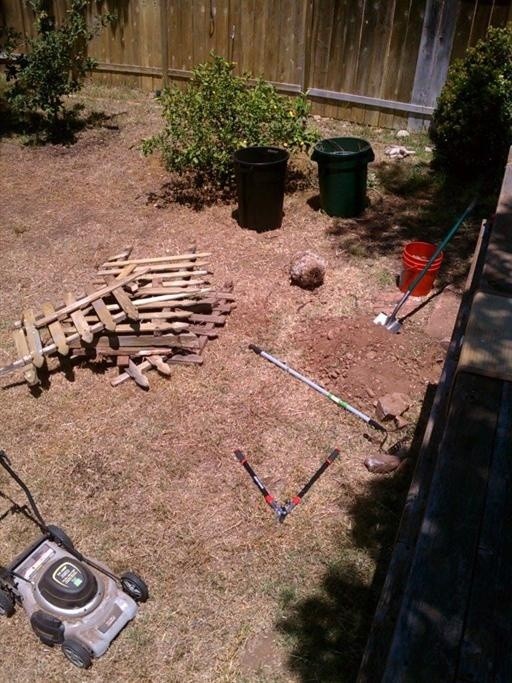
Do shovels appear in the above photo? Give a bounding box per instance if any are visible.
[372,200,478,333]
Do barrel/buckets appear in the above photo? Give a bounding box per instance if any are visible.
[399,240,444,297]
